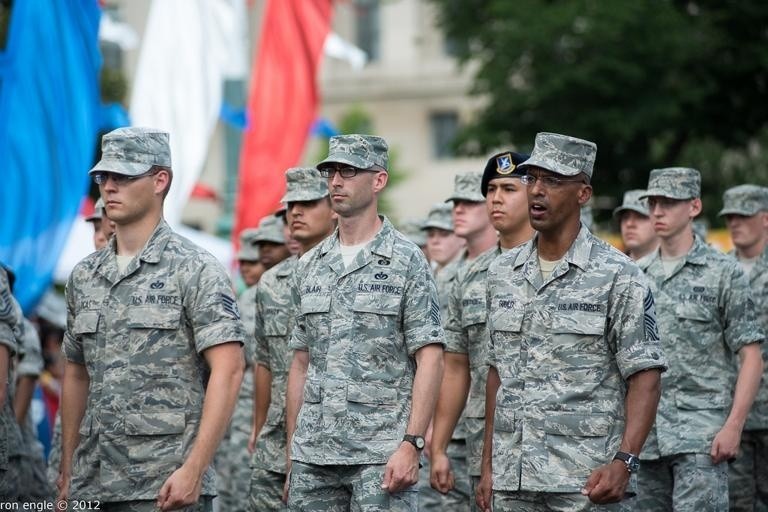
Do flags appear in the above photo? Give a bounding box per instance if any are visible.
[128,0,246,230]
[2,0,104,317]
[234,1,338,266]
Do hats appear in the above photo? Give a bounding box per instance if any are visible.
[279,166,330,203]
[315,134,390,171]
[88,126,172,177]
[85,197,104,221]
[420,204,454,232]
[638,167,702,199]
[444,170,486,203]
[250,214,284,244]
[236,228,260,262]
[398,218,427,247]
[516,133,597,179]
[613,189,650,217]
[274,205,288,216]
[481,152,529,197]
[716,181,767,217]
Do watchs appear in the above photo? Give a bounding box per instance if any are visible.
[402,434,426,451]
[612,451,641,473]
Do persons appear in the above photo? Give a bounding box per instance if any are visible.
[84,205,105,253]
[99,202,116,243]
[609,190,659,271]
[235,230,264,286]
[436,171,498,512]
[246,166,350,511]
[217,215,294,512]
[635,167,764,511]
[475,133,668,511]
[431,150,531,512]
[719,183,768,512]
[421,207,463,273]
[275,204,301,254]
[0,264,44,511]
[57,125,245,511]
[283,133,445,512]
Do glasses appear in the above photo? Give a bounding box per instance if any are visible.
[642,198,675,209]
[520,175,583,187]
[93,170,153,184]
[321,167,358,178]
